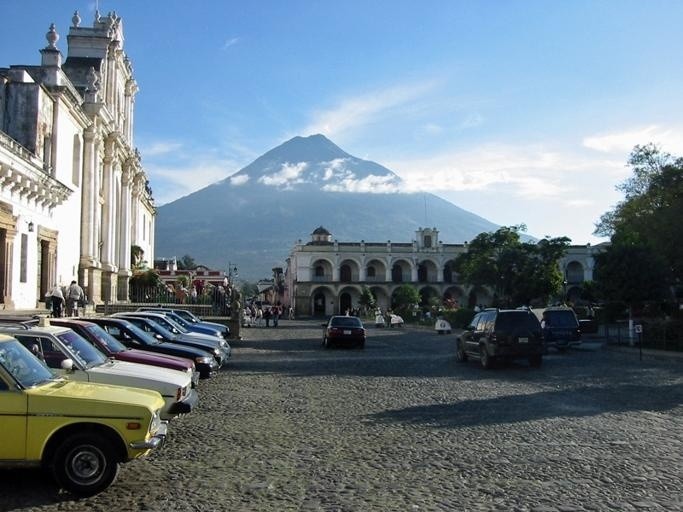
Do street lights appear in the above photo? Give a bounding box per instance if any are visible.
[562,280,568,305]
[499,272,505,302]
[227,263,238,287]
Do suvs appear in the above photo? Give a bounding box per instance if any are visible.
[455,306,542,368]
[253,299,281,316]
[526,303,583,353]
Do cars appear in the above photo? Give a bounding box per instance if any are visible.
[320,316,365,349]
[590,299,682,311]
[577,315,599,334]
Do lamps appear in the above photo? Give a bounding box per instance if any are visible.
[22,220,34,233]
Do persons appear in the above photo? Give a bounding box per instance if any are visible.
[45,286,65,318]
[66,281,83,317]
[346,306,393,327]
[246,303,294,327]
